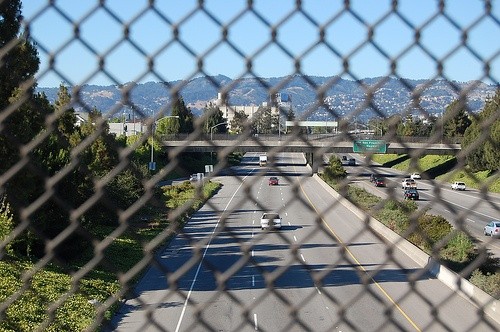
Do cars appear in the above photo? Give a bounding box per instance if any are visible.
[411,172,421,179]
[260,213,283,230]
[451,182,466,191]
[268,177,279,185]
[370,173,386,187]
[349,159,355,165]
[483,221,500,238]
[404,189,419,200]
[341,156,347,161]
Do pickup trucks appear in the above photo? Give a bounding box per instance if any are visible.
[401,178,417,189]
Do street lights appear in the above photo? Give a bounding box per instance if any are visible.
[210,121,228,164]
[150,115,182,170]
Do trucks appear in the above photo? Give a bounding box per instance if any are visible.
[259,154,267,167]
